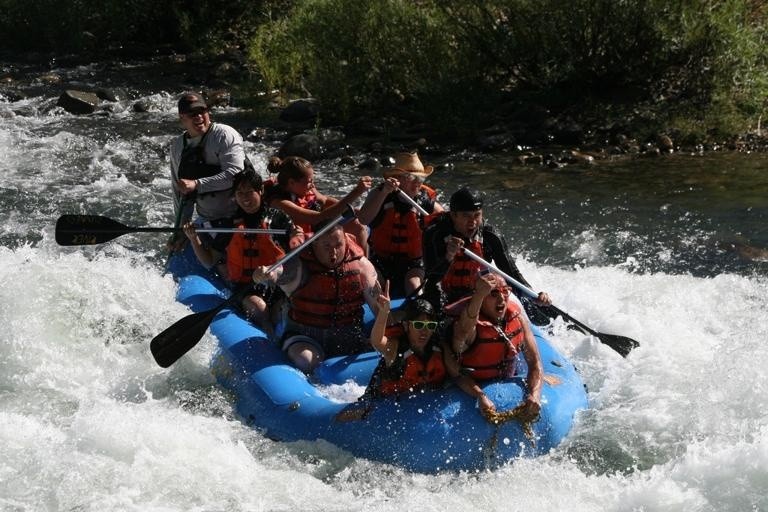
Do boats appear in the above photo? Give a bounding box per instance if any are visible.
[164,216,587,479]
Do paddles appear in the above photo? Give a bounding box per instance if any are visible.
[56,215,293,246]
[150,202,358,370]
[462,246,639,357]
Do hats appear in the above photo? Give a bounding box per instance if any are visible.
[449,185,483,212]
[383,153,433,178]
[178,93,209,113]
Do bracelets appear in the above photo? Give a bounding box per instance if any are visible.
[478,393,486,401]
[466,302,479,319]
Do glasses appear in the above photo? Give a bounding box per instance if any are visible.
[409,318,438,331]
[490,285,512,298]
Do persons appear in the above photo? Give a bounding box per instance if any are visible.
[165,92,255,255]
[251,218,392,372]
[264,157,372,233]
[421,186,553,309]
[362,279,447,401]
[450,268,543,419]
[359,152,443,278]
[182,170,307,332]
